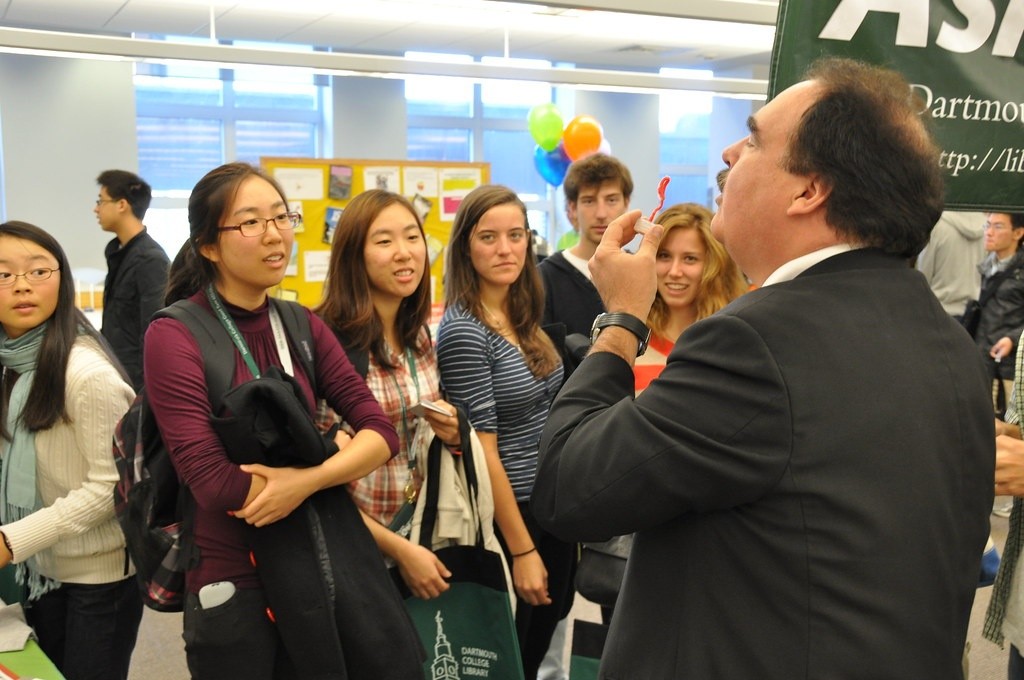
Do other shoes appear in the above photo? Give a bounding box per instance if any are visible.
[995,502,1016,518]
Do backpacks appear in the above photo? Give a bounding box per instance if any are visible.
[113,284,316,613]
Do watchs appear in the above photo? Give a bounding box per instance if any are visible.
[589,311,652,357]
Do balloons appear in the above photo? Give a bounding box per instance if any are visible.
[531,103,613,190]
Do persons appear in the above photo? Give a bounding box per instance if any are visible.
[915,211,987,323]
[981,328,1024,680]
[436,185,575,680]
[308,190,516,680]
[531,62,997,680]
[95,170,170,585]
[0,219,144,680]
[601,203,750,398]
[975,213,1024,421]
[535,153,634,376]
[145,162,427,680]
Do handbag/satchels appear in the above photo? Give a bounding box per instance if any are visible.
[960,299,983,335]
[414,408,525,680]
[575,524,634,605]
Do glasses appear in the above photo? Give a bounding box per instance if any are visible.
[98,196,123,206]
[0,266,61,289]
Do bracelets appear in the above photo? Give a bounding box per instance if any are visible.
[1,531,13,556]
[509,546,538,558]
